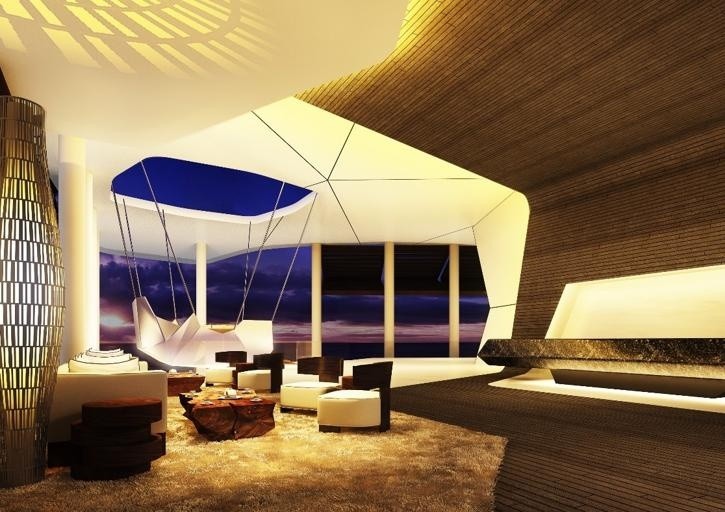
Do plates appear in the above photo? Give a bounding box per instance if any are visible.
[201,402,214,405]
[250,397,263,402]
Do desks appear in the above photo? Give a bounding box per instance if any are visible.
[178,392,273,439]
[167,374,205,396]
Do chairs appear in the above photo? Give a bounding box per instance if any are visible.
[317,361,392,432]
[236,352,283,390]
[205,351,247,387]
[279,356,344,415]
[46,348,167,467]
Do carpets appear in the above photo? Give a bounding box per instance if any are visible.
[0,390,510,512]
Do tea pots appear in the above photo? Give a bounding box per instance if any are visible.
[224,384,236,398]
